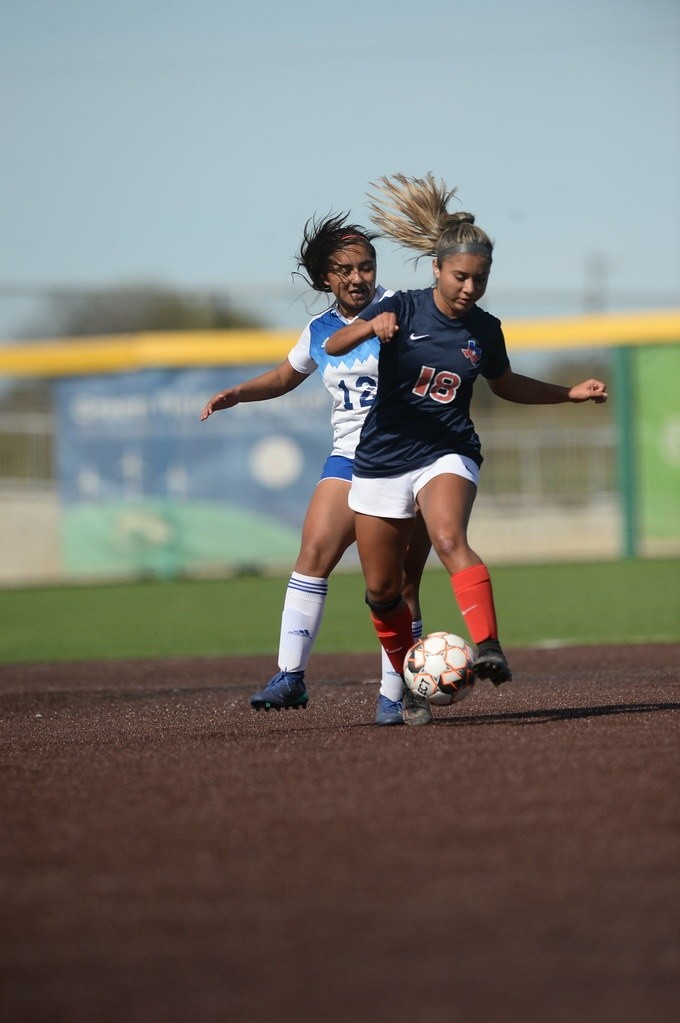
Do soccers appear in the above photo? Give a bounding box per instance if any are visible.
[404,630,479,708]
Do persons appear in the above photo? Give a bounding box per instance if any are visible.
[199,207,433,727]
[324,170,608,726]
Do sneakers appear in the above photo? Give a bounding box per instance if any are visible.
[375,694,403,727]
[471,647,514,687]
[401,685,432,726]
[249,670,308,712]
